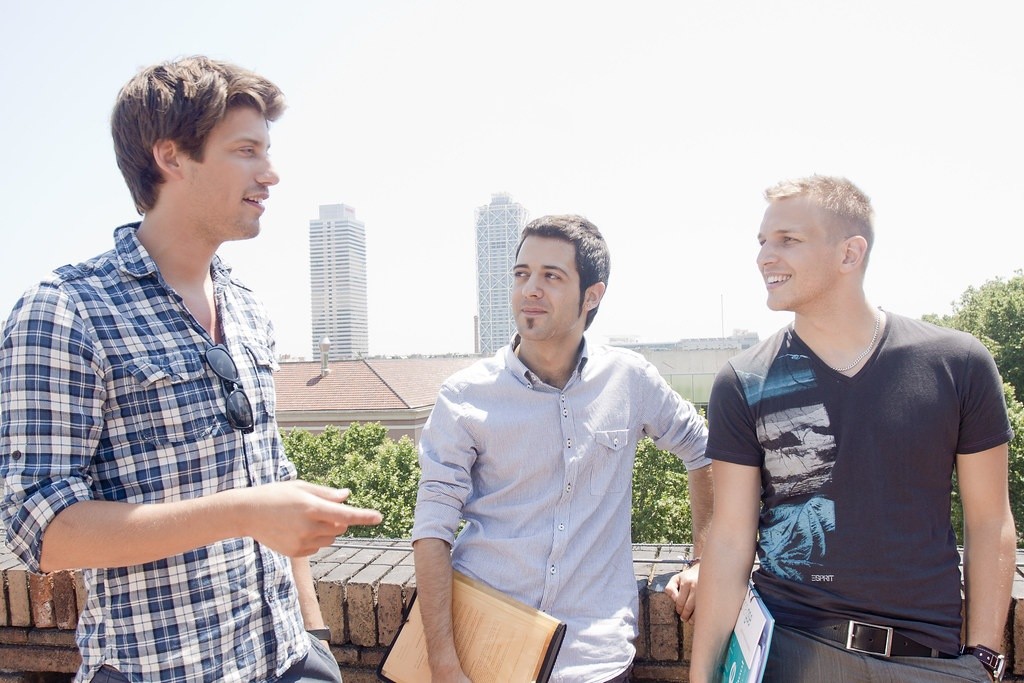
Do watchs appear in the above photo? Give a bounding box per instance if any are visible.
[960,644,1007,683]
[306,625,332,644]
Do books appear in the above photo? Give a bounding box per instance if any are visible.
[378,568,566,683]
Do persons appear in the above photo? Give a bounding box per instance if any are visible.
[412,211,719,683]
[0,54,382,683]
[689,174,1016,683]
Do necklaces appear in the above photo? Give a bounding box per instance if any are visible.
[832,304,881,371]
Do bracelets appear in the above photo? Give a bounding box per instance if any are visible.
[688,557,701,566]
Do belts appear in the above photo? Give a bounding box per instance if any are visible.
[764,603,960,660]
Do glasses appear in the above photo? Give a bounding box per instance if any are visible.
[204,344,255,433]
